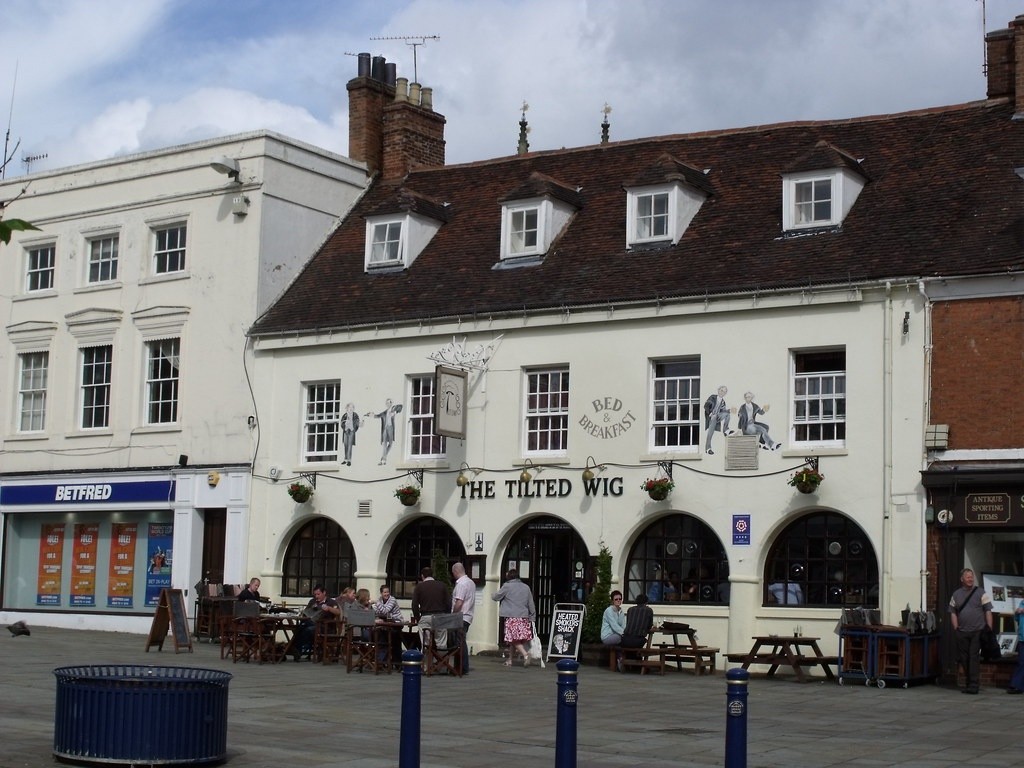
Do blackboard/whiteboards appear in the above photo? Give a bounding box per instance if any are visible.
[146,588,192,647]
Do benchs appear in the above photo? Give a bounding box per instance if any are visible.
[723,653,844,666]
[592,643,719,673]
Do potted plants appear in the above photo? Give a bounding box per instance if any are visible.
[579,539,617,666]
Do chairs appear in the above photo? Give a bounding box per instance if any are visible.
[216,599,465,678]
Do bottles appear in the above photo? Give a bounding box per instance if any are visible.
[266,601,271,612]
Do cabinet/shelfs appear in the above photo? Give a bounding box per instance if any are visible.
[200,596,232,636]
[844,625,936,677]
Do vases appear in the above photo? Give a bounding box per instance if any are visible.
[292,493,309,503]
[400,494,418,506]
[797,479,817,494]
[648,487,669,501]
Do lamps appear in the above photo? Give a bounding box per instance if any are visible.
[458,461,480,486]
[582,456,607,481]
[521,458,541,482]
[212,155,244,183]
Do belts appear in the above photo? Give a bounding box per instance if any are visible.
[421,611,446,616]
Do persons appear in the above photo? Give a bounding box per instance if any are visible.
[294,584,340,659]
[768,568,804,605]
[600,590,653,671]
[690,564,711,601]
[338,587,383,642]
[948,568,993,693]
[492,569,536,666]
[411,567,451,676]
[238,578,261,603]
[649,567,674,604]
[374,585,404,630]
[1007,599,1024,694]
[451,562,476,673]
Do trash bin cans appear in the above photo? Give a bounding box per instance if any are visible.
[51,664,234,768]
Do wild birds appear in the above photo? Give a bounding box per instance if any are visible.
[6,620,32,637]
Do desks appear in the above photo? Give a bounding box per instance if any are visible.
[741,637,834,682]
[358,622,419,675]
[258,614,311,663]
[640,627,702,674]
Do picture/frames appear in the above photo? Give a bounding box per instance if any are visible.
[998,632,1019,655]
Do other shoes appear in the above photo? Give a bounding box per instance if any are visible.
[502,658,512,666]
[450,666,469,675]
[523,654,530,667]
[617,656,626,671]
[961,688,978,694]
[1007,688,1023,694]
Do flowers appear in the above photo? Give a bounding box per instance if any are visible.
[287,483,313,496]
[392,485,421,498]
[788,468,824,487]
[640,477,675,492]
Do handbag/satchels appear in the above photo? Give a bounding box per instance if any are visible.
[522,621,542,660]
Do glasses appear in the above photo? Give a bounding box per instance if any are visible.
[615,599,622,601]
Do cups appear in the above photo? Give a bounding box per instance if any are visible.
[387,613,393,620]
[299,606,304,613]
[411,617,415,623]
